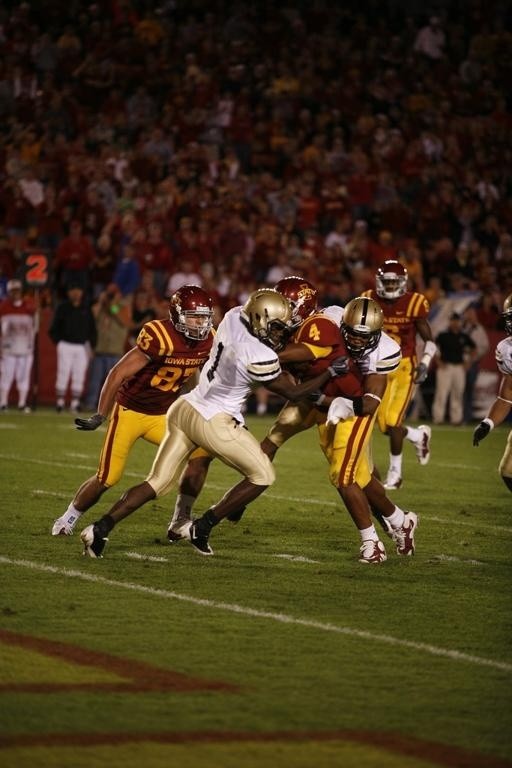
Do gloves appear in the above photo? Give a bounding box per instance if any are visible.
[329,355,354,378]
[413,362,428,384]
[472,422,490,446]
[75,414,107,431]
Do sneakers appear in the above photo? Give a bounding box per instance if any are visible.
[381,471,403,490]
[51,517,74,536]
[167,504,246,555]
[414,425,431,465]
[358,508,419,565]
[80,522,109,559]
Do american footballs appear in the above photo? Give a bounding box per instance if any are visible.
[283,369,302,386]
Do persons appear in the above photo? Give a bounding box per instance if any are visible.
[313,297,417,566]
[0,0,511,424]
[81,289,352,559]
[473,294,512,492]
[362,259,437,491]
[269,277,418,565]
[51,285,217,540]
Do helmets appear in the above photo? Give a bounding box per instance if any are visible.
[168,284,214,343]
[239,276,319,352]
[375,260,409,300]
[501,295,512,337]
[338,297,385,359]
